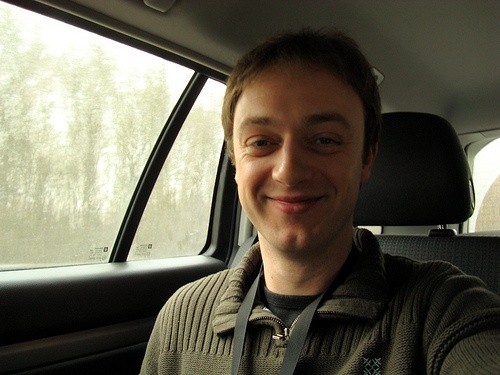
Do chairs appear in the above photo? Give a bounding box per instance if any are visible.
[359,110,499,294]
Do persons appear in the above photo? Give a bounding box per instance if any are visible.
[139,28,500,375]
